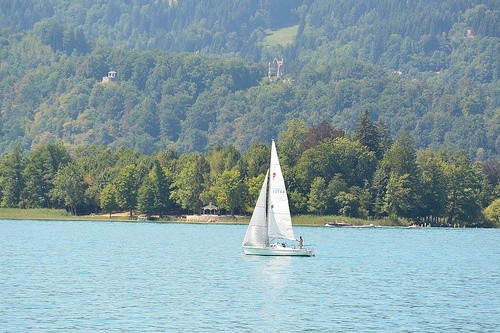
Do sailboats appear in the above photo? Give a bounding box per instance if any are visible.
[243,140,312,256]
